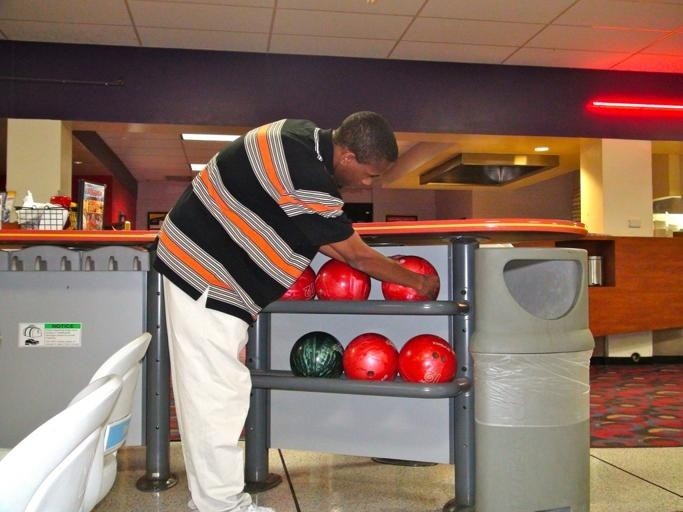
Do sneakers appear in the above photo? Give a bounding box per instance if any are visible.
[239,501,272,512]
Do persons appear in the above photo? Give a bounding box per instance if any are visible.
[149,110,440,512]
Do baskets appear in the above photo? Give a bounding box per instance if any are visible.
[14,206,81,229]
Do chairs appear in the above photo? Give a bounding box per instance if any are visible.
[1,375,122,508]
[86,331,152,506]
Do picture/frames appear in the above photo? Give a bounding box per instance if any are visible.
[385,215,418,222]
[146,211,167,230]
